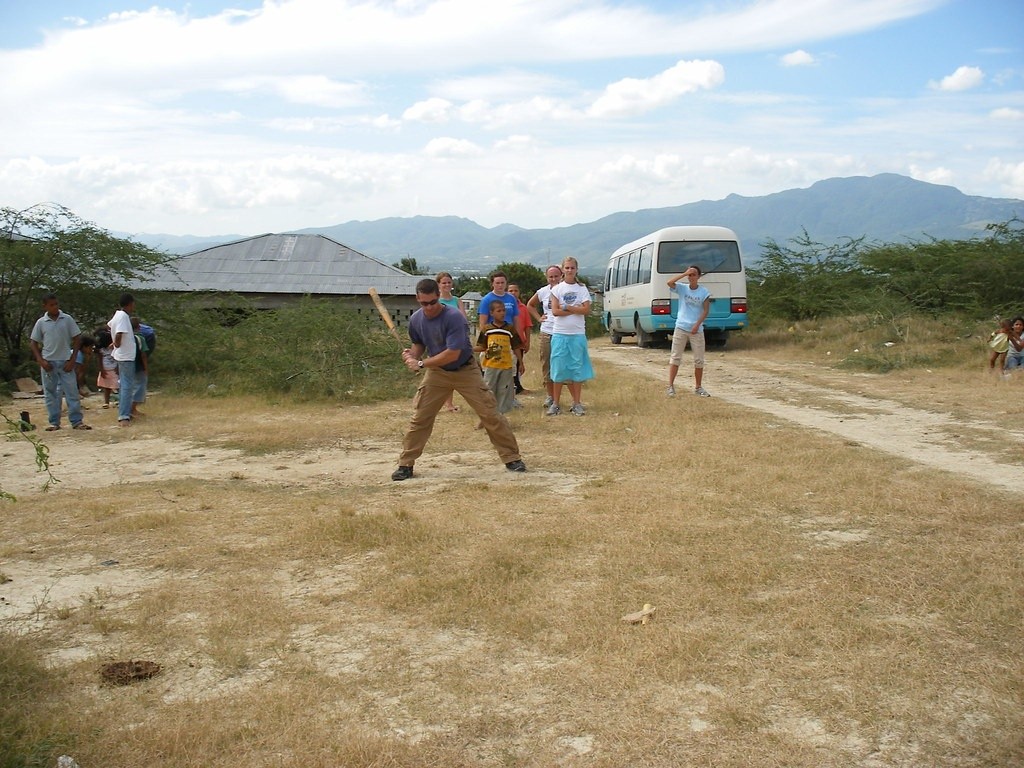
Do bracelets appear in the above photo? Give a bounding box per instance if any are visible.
[562,304,567,311]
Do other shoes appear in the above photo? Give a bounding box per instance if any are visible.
[448,405,459,411]
[113,403,121,408]
[75,423,92,430]
[45,425,61,431]
[102,403,110,409]
[132,410,145,416]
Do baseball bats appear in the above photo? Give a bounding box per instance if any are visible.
[368,286,421,377]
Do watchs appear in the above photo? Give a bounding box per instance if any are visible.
[418,360,424,368]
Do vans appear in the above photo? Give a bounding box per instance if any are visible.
[597,225,748,347]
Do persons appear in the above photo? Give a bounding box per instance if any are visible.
[391,279,527,481]
[527,266,564,406]
[70,317,156,416]
[106,293,137,426]
[477,272,533,394]
[986,316,1024,375]
[666,266,710,398]
[545,256,594,416]
[29,294,91,431]
[472,300,525,428]
[435,272,467,412]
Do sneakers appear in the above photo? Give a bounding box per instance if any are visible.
[572,405,585,416]
[392,466,413,480]
[546,404,560,415]
[542,396,553,406]
[506,461,524,471]
[695,387,711,397]
[668,386,676,398]
